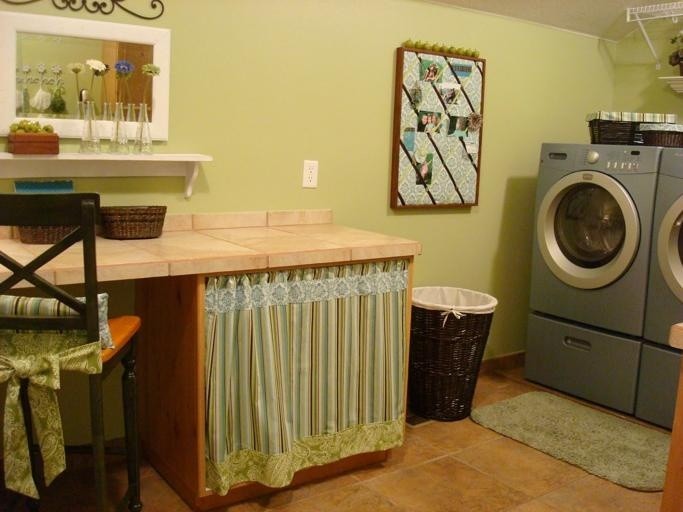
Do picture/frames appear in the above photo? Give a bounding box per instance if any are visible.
[389,47,488,211]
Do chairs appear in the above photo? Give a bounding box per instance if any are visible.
[0,192,144,512]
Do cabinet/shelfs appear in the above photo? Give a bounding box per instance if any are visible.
[139,257,416,512]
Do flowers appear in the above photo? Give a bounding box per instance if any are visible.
[86,56,160,102]
[68,61,132,102]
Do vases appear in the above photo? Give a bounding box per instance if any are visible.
[82,101,155,152]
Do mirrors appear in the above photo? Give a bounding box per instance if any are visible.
[0,10,171,141]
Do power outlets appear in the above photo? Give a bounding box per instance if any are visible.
[302,159,318,187]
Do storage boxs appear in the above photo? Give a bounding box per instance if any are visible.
[584,110,683,146]
[17,205,166,245]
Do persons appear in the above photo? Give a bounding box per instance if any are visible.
[430,113,439,128]
[418,112,428,133]
[423,113,435,133]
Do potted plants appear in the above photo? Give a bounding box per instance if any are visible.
[7,120,61,155]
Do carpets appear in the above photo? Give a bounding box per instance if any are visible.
[470,390,671,493]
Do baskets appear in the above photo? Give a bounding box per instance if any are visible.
[639,123,683,148]
[586,111,676,145]
[102,205,167,239]
[18,223,75,244]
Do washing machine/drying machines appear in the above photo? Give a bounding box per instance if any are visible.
[523,140,661,417]
[634,147,682,432]
